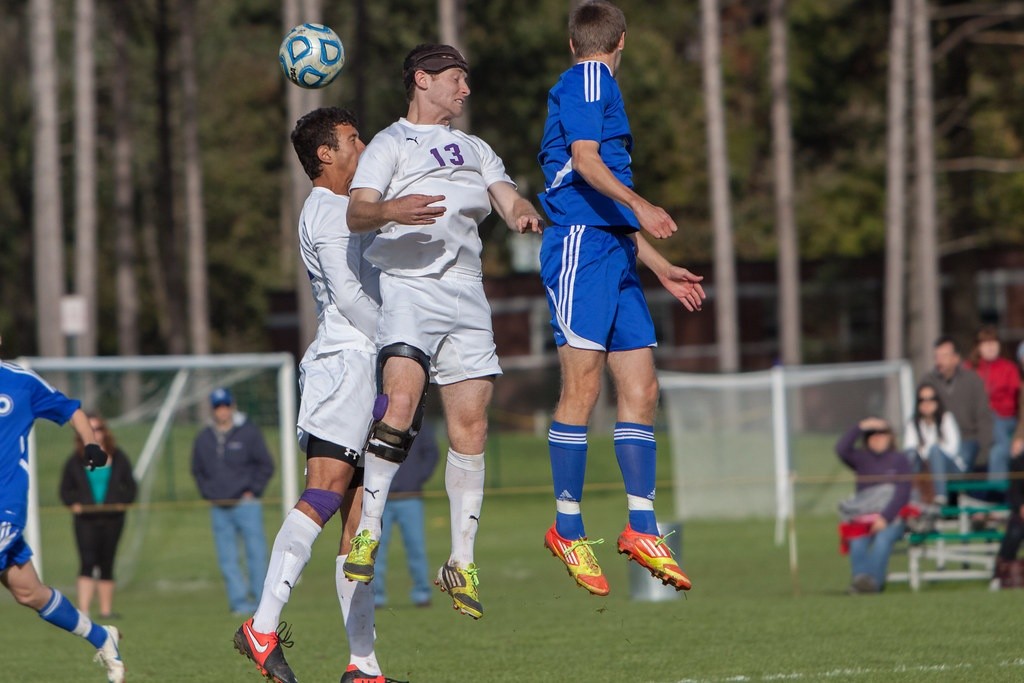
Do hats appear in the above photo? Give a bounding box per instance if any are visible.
[209,386,232,408]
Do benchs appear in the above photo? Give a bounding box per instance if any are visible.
[889,476,1013,591]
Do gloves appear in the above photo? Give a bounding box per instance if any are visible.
[81,444,108,472]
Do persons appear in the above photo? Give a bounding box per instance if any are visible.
[836,417,912,592]
[59,413,138,616]
[372,419,438,606]
[901,330,1024,589]
[230,107,408,683]
[191,388,273,615]
[343,43,545,618]
[0,359,127,683]
[538,1,706,596]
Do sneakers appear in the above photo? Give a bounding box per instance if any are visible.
[341,664,411,682]
[543,524,609,601]
[341,530,380,586]
[614,521,693,594]
[433,561,484,621]
[92,624,124,683]
[231,618,302,683]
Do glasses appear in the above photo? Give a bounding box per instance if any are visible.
[212,403,231,410]
[918,397,936,402]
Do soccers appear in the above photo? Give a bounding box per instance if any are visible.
[278,23,345,89]
